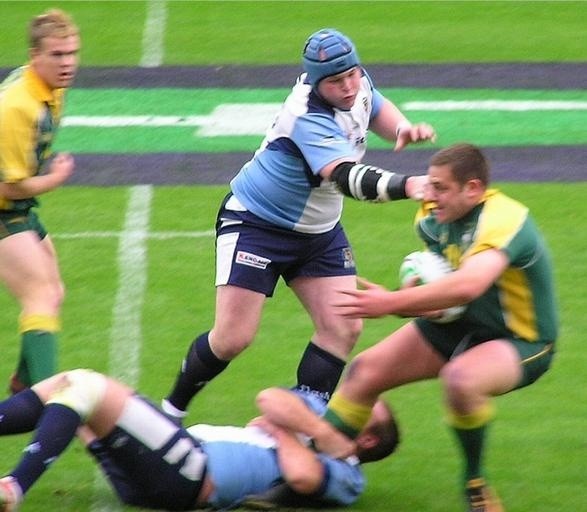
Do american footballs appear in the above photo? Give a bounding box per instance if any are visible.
[400,249,470,323]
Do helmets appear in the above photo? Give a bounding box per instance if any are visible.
[302,28,359,85]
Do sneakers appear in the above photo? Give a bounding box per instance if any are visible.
[466,479,502,512]
[249,482,314,510]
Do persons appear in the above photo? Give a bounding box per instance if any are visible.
[242,140,562,511]
[1,8,82,396]
[159,27,438,425]
[0,365,397,512]
